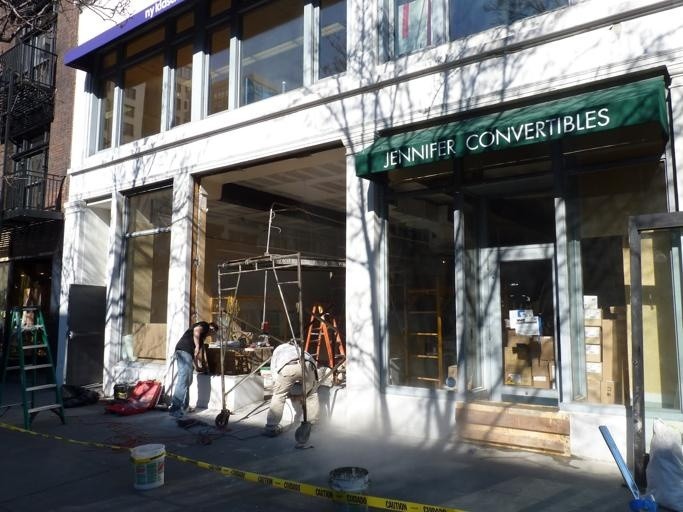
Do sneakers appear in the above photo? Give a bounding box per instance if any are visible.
[170,407,195,419]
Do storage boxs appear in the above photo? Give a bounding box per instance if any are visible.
[502,295,625,404]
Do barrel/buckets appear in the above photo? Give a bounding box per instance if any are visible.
[130,442,166,490]
[259,367,274,388]
[327,466,369,511]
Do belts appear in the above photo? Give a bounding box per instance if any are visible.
[287,361,300,365]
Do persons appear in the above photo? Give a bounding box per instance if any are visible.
[264,342,317,438]
[167,321,219,418]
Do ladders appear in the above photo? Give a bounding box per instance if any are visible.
[304,302,346,370]
[403,277,444,390]
[0,305,66,430]
